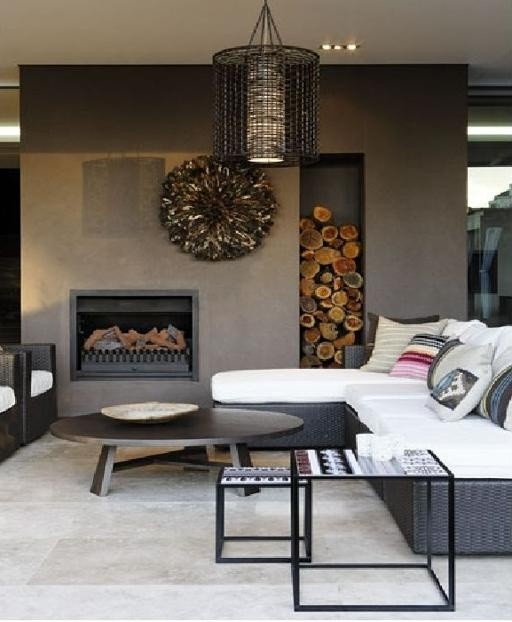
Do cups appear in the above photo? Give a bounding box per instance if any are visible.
[355,432,405,463]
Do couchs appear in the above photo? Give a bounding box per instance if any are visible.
[212,369,511,556]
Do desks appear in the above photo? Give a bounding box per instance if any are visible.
[217,448,456,612]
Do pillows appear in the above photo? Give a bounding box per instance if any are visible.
[363,312,511,434]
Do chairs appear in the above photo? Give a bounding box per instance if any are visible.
[0,341,56,464]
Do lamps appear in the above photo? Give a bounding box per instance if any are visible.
[211,0,320,168]
